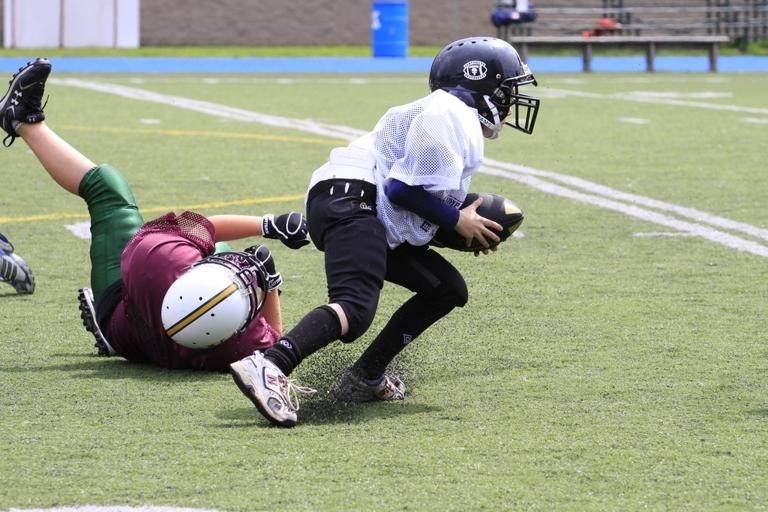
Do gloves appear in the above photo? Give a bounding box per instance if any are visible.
[244,244,282,293]
[261,212,310,249]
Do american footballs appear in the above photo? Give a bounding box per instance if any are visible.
[435,192,524,252]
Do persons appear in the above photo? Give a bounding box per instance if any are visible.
[4,57,308,369]
[228,31,540,430]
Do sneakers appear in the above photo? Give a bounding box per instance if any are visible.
[0,57,51,147]
[229,349,317,429]
[328,367,405,402]
[77,287,118,357]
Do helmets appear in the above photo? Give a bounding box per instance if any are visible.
[160,251,269,350]
[428,35,540,134]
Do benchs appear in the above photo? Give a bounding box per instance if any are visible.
[490,0,768,76]
